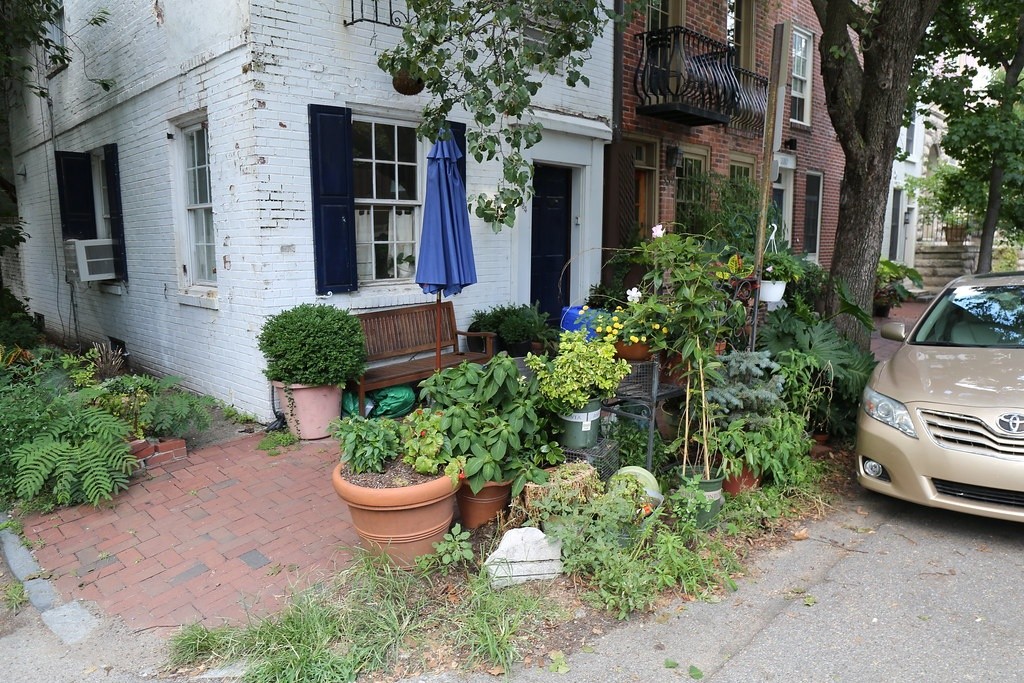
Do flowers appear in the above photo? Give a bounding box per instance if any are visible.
[740,251,806,285]
[626,224,756,340]
[574,302,679,344]
[324,406,466,481]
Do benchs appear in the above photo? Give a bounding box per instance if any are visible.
[346,301,496,420]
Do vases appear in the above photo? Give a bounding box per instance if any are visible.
[611,338,660,361]
[332,453,462,577]
[704,337,727,355]
[757,279,788,302]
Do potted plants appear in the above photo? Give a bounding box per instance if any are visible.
[586,476,666,553]
[870,256,925,318]
[691,349,815,499]
[672,284,728,531]
[254,300,368,441]
[521,461,603,522]
[417,350,565,533]
[526,328,633,450]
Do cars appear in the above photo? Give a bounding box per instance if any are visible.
[855,271,1024,522]
